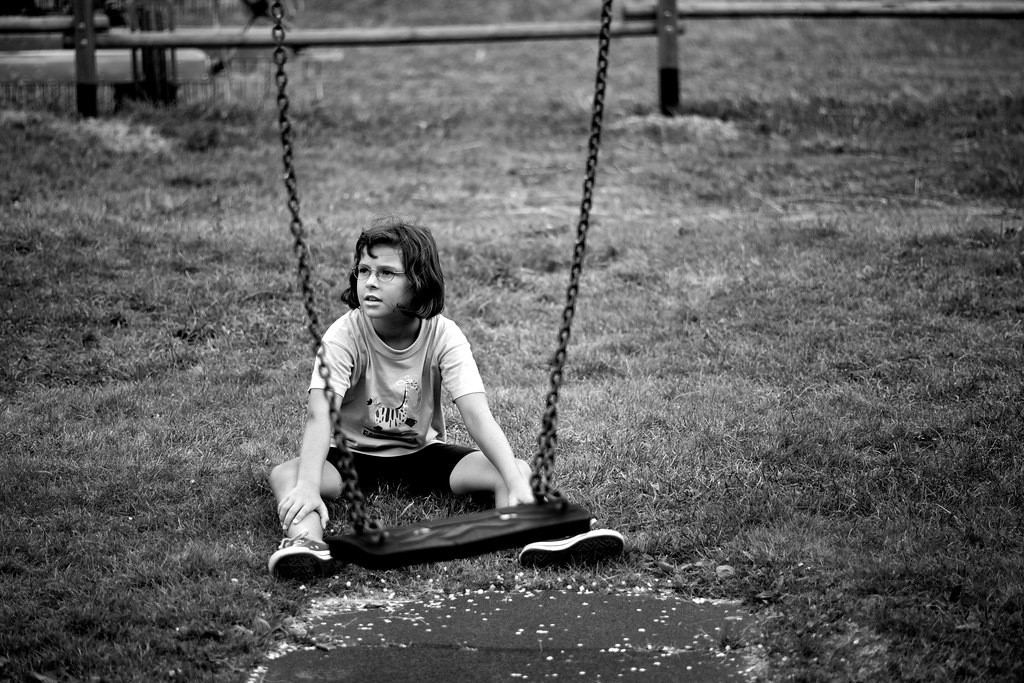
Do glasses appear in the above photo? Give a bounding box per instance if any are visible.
[351,264,406,282]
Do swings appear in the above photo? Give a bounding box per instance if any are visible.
[271,2,611,567]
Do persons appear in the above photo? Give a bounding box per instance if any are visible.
[267,210,624,581]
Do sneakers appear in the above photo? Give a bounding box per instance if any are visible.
[269,534,336,581]
[519,529,625,568]
